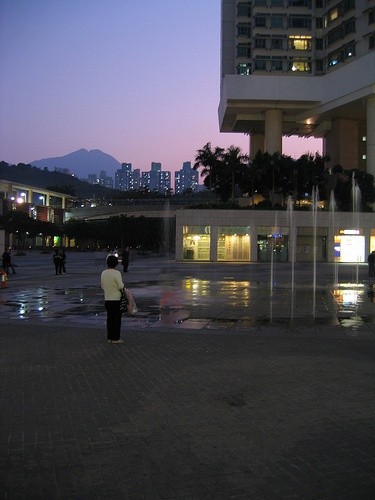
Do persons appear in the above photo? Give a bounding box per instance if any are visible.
[105,246,129,273]
[368,251,375,276]
[53,249,66,275]
[101,256,126,344]
[0,248,16,288]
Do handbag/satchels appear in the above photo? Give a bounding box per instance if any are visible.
[119,288,128,312]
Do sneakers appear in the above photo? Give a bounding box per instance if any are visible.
[112,340,124,343]
[108,340,112,343]
